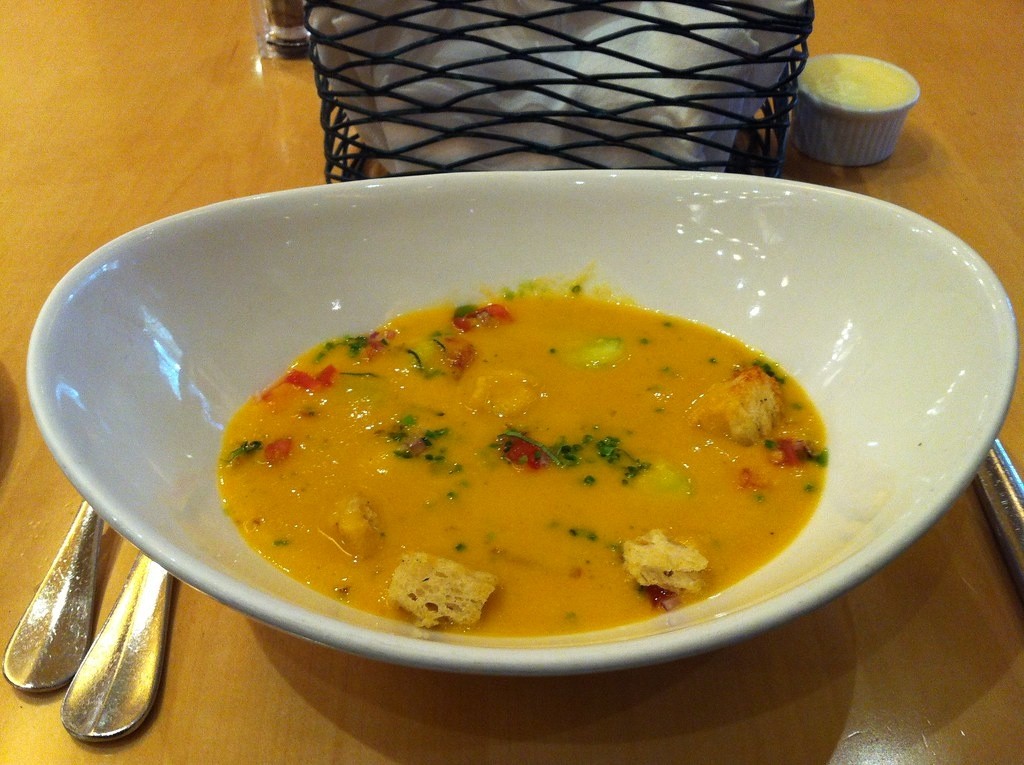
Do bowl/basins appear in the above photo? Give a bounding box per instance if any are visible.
[25,169,1018,675]
[791,54,920,166]
